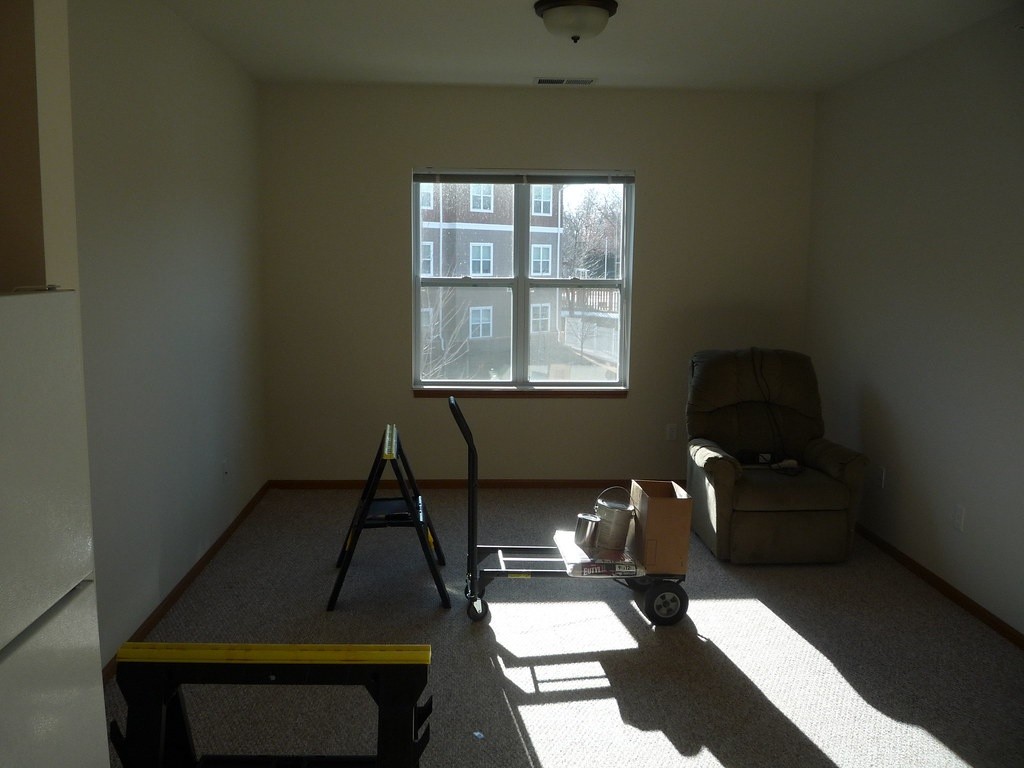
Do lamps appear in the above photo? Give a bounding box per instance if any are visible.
[533,0,619,43]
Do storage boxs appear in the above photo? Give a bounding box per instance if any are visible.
[626,479,693,574]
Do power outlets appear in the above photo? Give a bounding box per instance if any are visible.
[666,424,677,440]
[954,504,965,532]
[881,466,886,484]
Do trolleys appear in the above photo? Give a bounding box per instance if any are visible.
[448,395,689,626]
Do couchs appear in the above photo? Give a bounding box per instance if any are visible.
[684,347,883,565]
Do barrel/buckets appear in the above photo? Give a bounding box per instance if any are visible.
[575,513,601,548]
[594,486,635,550]
